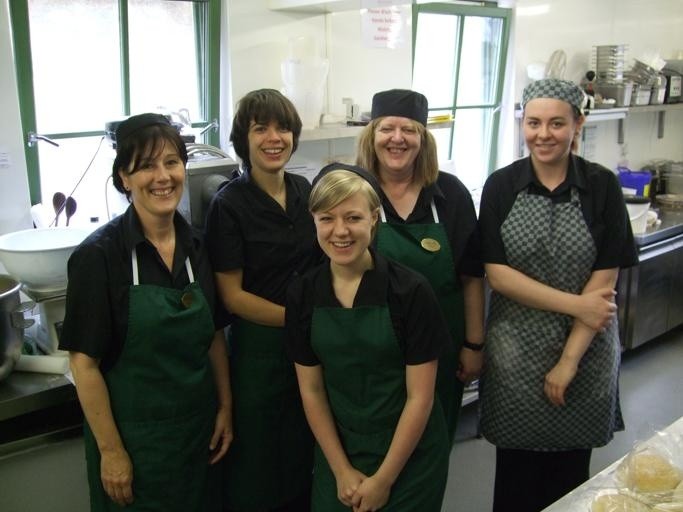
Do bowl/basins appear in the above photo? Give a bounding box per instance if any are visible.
[656,193,683,211]
[0,227,89,289]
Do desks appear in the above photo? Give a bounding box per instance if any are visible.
[539,415,683,512]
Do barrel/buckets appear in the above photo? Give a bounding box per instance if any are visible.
[623,197,652,234]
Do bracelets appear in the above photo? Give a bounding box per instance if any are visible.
[463,339,485,351]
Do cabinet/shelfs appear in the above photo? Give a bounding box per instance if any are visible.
[618,224,683,350]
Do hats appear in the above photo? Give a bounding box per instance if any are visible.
[370,86,430,128]
[521,76,588,118]
[114,111,172,144]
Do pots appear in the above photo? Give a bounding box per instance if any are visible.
[0,275,36,382]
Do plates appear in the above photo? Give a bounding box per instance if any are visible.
[594,103,614,108]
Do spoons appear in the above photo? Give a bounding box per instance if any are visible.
[53,192,66,227]
[66,197,77,227]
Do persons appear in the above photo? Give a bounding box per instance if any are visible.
[207,88,322,512]
[282,163,451,511]
[57,112,234,512]
[356,90,488,457]
[478,80,640,512]
